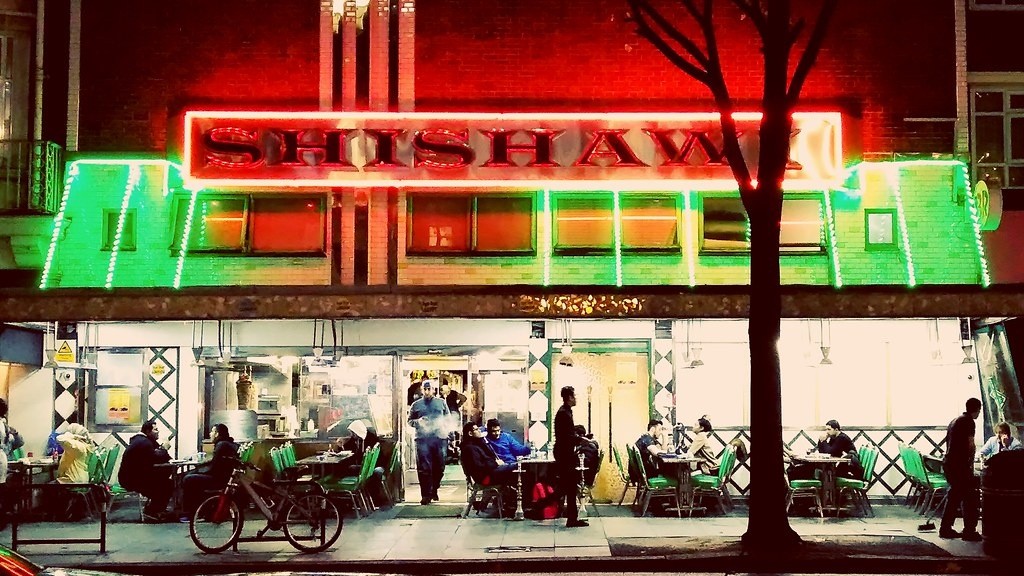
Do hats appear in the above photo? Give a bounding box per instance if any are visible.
[422,380,431,388]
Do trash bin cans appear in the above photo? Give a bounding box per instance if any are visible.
[980,450,1024,561]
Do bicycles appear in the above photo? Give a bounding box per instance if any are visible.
[189,439,343,554]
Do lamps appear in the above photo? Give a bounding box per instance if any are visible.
[186,321,236,371]
[39,322,97,372]
[679,319,705,370]
[923,319,978,366]
[801,318,833,366]
[311,320,345,367]
[559,320,575,366]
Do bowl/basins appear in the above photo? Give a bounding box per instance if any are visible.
[820,454,830,459]
[537,452,546,456]
[341,451,352,456]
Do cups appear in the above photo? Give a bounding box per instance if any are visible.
[193,454,198,461]
[668,434,673,445]
[811,449,816,453]
[45,456,52,459]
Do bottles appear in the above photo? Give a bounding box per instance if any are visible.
[28,452,33,462]
[531,444,535,457]
[54,450,58,460]
[262,426,268,438]
[18,457,24,463]
[548,445,553,458]
[975,453,987,470]
[308,419,315,432]
[198,451,207,462]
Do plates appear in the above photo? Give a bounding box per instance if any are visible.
[805,455,817,458]
[169,460,187,463]
[329,444,332,452]
[38,460,53,463]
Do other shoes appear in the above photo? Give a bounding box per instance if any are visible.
[566,520,589,527]
[143,509,159,520]
[421,497,429,505]
[532,501,545,520]
[962,532,983,542]
[940,527,962,538]
[432,490,439,502]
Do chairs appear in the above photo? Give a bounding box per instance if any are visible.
[52,436,964,518]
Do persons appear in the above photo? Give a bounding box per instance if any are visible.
[818,419,863,505]
[574,424,599,489]
[35,423,92,516]
[627,419,678,506]
[668,414,720,504]
[407,376,430,405]
[978,422,1022,459]
[460,422,525,520]
[117,420,173,519]
[485,418,538,465]
[0,398,13,454]
[407,380,451,505]
[938,397,983,541]
[336,420,385,506]
[180,424,238,522]
[536,387,590,527]
[443,378,467,464]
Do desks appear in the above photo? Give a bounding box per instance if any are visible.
[925,454,985,519]
[790,452,853,516]
[155,455,213,518]
[7,462,59,517]
[657,453,710,514]
[298,449,356,513]
[515,453,558,510]
